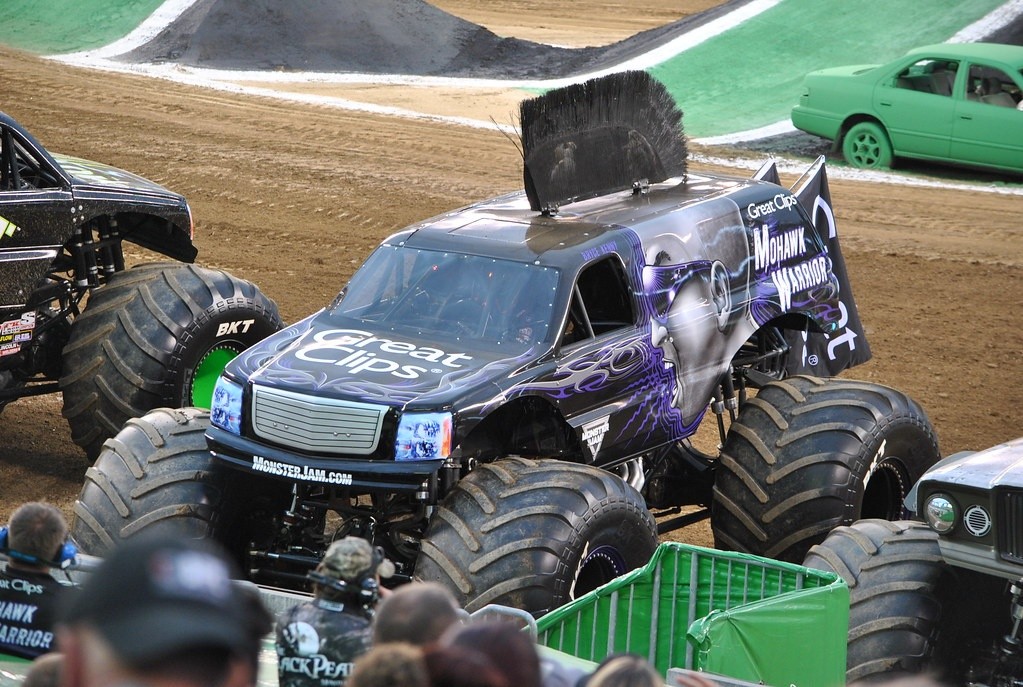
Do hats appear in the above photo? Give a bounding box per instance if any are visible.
[314,536,395,582]
[75,533,250,667]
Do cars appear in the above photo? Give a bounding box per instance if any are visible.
[790,43,1023,171]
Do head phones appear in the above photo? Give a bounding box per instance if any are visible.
[0,524,78,570]
[305,569,380,605]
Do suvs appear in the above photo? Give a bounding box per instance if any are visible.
[0,110,283,465]
[69,167,945,627]
[799,435,1023,687]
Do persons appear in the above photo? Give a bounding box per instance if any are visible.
[375,580,461,648]
[55,533,245,686]
[22,652,65,687]
[425,643,507,687]
[458,625,541,687]
[0,501,79,661]
[348,642,428,687]
[543,279,594,347]
[230,580,275,687]
[588,656,664,687]
[274,535,395,687]
